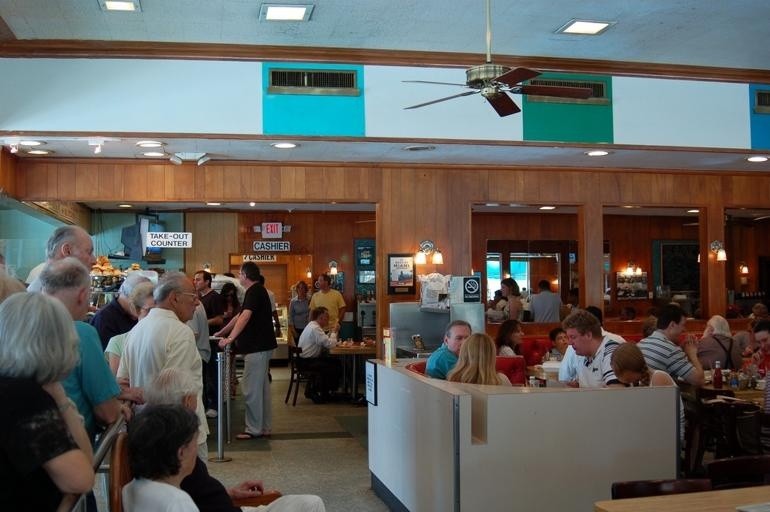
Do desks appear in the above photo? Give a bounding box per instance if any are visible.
[593,486,770,512]
[681,382,765,411]
[328,341,377,402]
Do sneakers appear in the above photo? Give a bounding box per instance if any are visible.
[205,409,218,419]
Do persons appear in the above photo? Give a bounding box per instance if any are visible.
[288,275,346,399]
[91,261,275,464]
[731,303,770,379]
[495,319,524,357]
[485,277,565,323]
[119,365,324,511]
[633,300,742,463]
[550,305,686,444]
[1,223,144,512]
[425,320,512,387]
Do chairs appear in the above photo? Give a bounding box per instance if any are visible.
[284,346,308,407]
[109,432,134,512]
[708,455,770,490]
[612,479,709,499]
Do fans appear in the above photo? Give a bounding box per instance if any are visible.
[403,0,592,118]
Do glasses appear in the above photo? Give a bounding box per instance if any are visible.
[178,291,199,300]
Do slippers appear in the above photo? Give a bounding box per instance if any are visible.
[233,430,272,440]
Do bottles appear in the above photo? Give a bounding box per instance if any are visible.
[705,360,765,391]
[509,288,533,322]
[656,283,671,300]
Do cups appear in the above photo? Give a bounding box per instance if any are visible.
[336,336,374,348]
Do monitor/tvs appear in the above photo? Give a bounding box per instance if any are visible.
[121,217,168,260]
